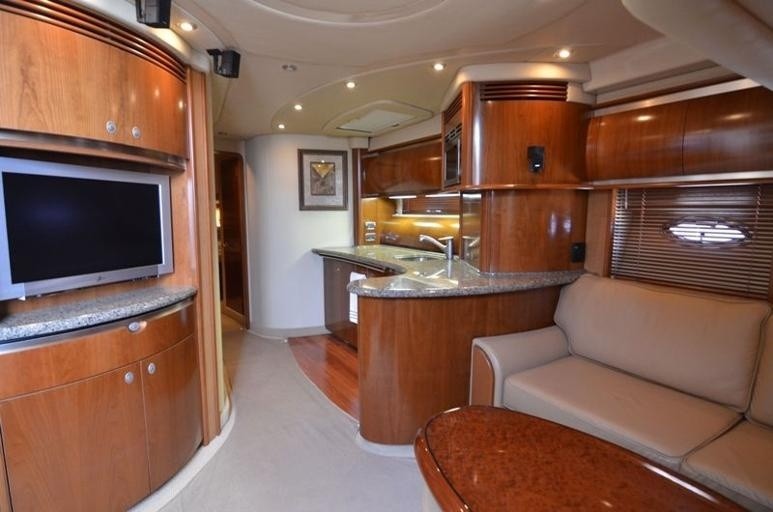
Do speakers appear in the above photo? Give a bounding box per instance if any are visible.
[221,50,241,78]
[144,0,171,28]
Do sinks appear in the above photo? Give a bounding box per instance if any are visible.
[394,253,445,262]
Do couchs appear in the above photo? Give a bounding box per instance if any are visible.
[466,270,772,512]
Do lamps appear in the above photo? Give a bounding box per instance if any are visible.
[132,0,173,29]
[205,45,241,80]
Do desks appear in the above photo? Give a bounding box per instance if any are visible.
[413,402,753,512]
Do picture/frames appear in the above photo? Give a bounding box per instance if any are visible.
[295,147,350,213]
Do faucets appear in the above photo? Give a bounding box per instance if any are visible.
[418,233,454,260]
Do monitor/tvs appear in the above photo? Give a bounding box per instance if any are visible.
[0,156,175,302]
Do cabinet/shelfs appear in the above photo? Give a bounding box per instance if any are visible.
[581,74,772,191]
[356,264,399,281]
[361,137,446,201]
[0,1,195,177]
[319,257,353,345]
[1,291,212,510]
